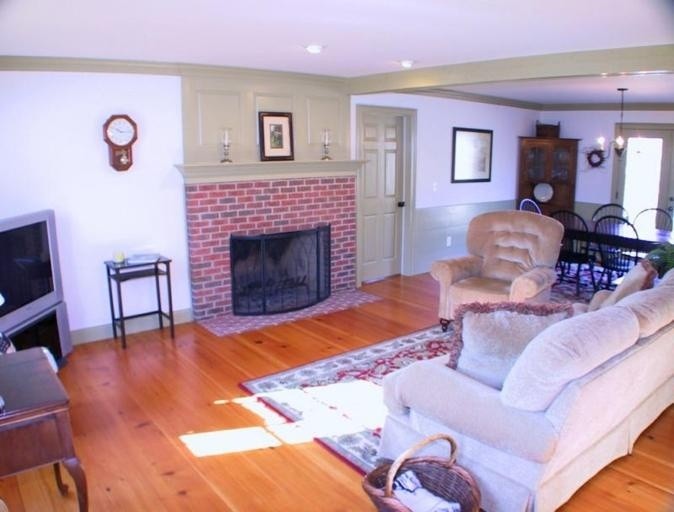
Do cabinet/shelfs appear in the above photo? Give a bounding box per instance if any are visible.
[104,254,177,350]
[2,346,89,512]
[515,135,581,228]
[521,199,542,214]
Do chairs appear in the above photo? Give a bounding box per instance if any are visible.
[635,207,673,230]
[595,216,638,290]
[548,210,594,295]
[591,204,630,223]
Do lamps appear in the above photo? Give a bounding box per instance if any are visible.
[594,88,647,157]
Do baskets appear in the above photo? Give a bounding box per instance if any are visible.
[361,433,481,512]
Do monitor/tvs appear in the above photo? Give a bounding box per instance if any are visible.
[0,209,64,333]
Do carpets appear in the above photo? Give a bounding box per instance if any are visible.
[239,323,460,478]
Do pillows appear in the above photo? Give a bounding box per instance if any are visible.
[447,301,573,389]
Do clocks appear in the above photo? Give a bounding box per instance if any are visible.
[104,114,138,171]
[534,182,554,203]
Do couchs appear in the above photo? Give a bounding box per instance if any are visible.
[375,258,674,512]
[431,210,566,321]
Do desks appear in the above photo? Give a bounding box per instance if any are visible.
[558,217,674,253]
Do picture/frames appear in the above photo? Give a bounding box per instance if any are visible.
[259,111,295,160]
[451,127,493,184]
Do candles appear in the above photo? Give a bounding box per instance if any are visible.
[323,129,332,146]
[222,130,231,145]
